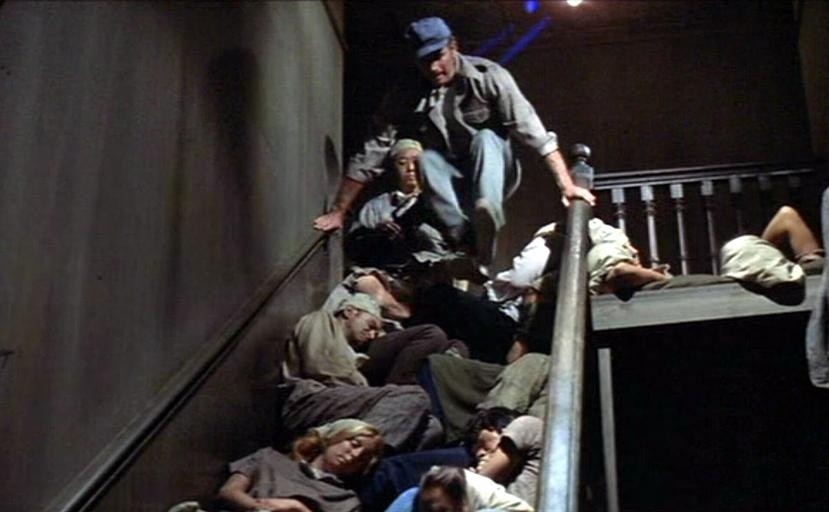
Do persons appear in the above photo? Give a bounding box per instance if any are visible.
[485,218,667,300]
[762,205,824,272]
[172,417,384,512]
[312,16,596,285]
[274,292,444,466]
[351,139,562,362]
[365,328,550,512]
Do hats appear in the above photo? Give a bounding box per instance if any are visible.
[390,138,423,157]
[405,16,452,59]
[334,292,384,320]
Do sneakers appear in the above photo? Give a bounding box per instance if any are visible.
[444,197,497,277]
